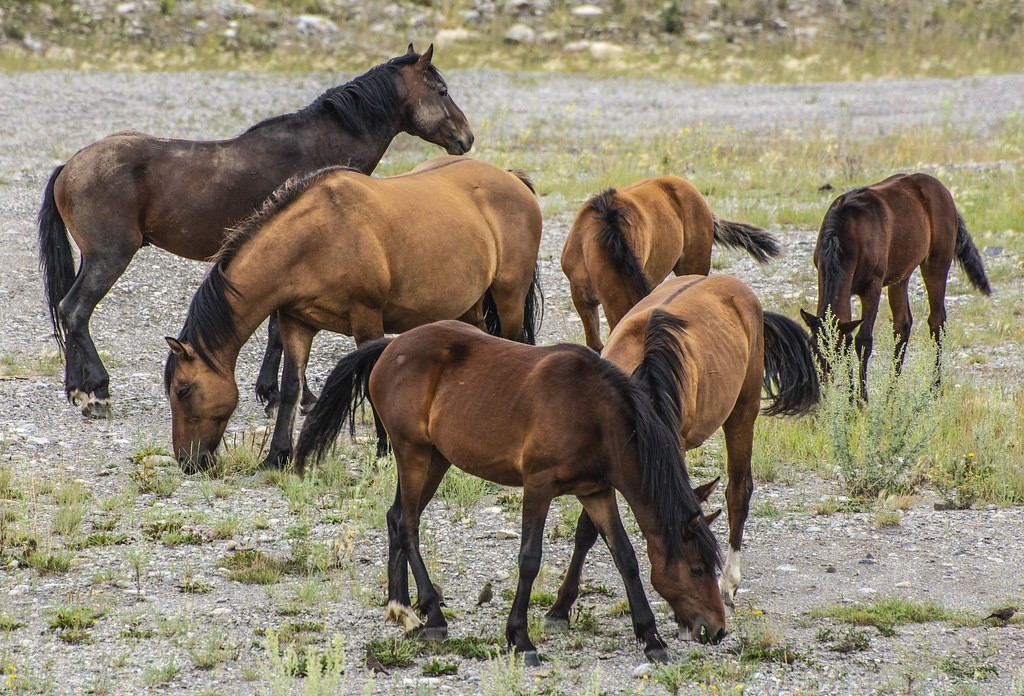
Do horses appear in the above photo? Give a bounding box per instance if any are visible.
[800,173,992,408]
[562,176,780,353]
[166,155,543,478]
[290,278,820,667]
[38,42,476,420]
